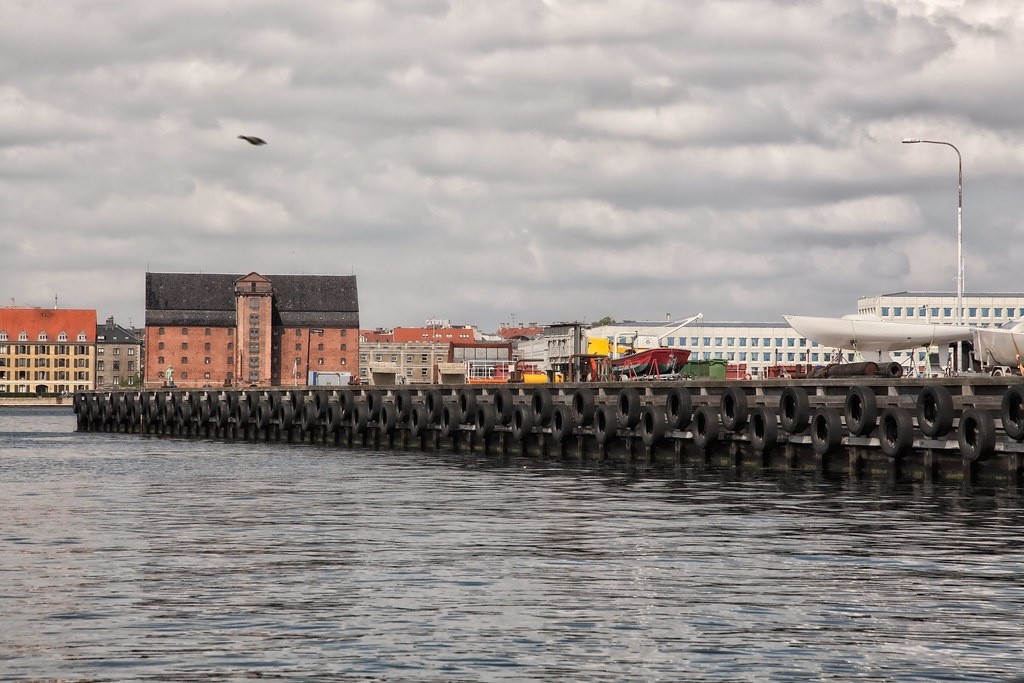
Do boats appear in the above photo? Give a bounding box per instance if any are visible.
[464,313,703,387]
[974,315,1024,369]
[782,313,977,353]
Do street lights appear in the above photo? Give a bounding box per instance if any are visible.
[902,138,963,375]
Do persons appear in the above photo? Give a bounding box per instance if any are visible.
[166,365,174,386]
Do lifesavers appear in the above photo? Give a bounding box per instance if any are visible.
[585,358,598,381]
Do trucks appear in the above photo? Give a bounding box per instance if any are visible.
[307,370,361,387]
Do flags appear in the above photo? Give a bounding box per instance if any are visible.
[293,360,296,375]
